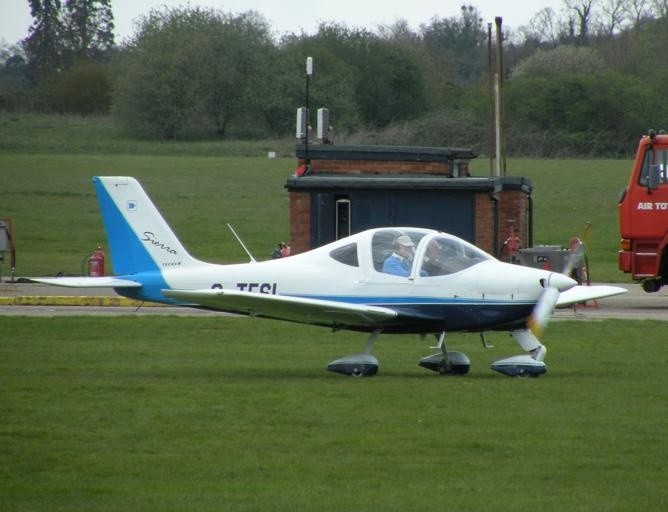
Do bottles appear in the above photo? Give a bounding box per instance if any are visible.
[396,235,414,247]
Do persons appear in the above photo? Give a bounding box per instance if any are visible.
[422,240,451,273]
[272,239,291,258]
[382,235,429,277]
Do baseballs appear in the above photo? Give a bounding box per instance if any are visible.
[82,246,105,277]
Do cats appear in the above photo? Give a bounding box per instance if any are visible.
[0,172,627,378]
